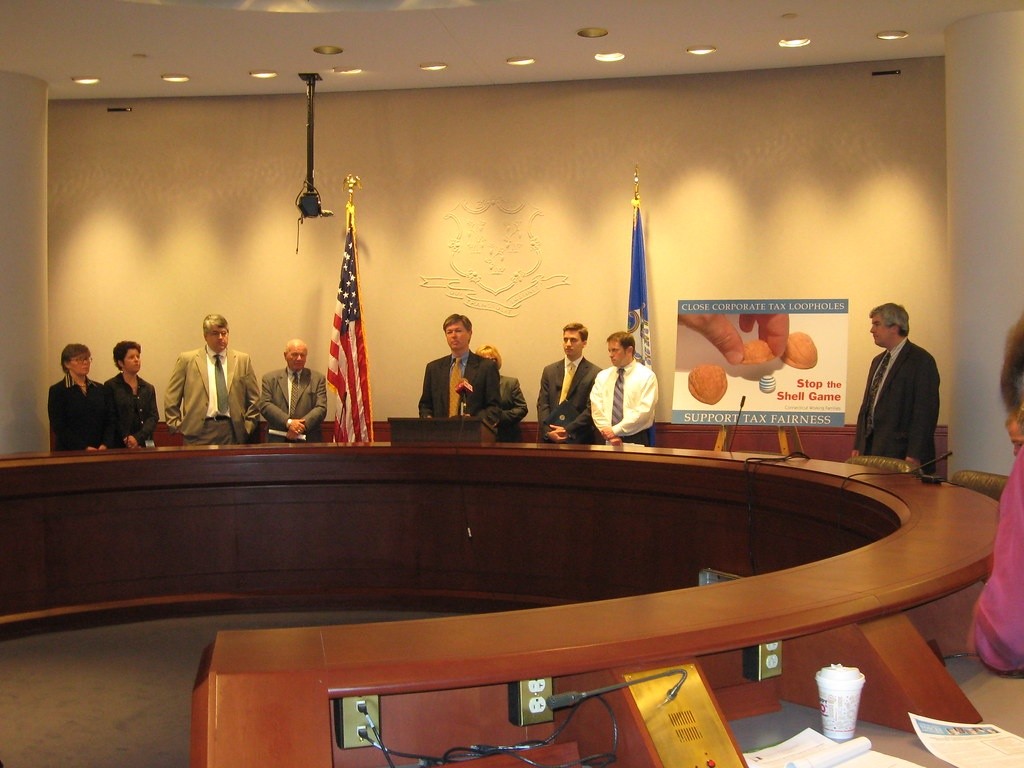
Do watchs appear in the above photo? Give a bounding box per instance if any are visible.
[287,418,293,428]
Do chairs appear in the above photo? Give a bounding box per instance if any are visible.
[844,455,924,476]
[951,470,1009,502]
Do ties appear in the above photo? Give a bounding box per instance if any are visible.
[862,352,890,439]
[612,369,626,426]
[558,364,573,406]
[213,354,229,414]
[448,358,462,417]
[290,372,298,419]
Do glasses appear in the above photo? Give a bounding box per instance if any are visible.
[70,356,92,364]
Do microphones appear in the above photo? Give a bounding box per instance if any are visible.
[336,392,348,446]
[536,403,542,444]
[729,396,746,451]
[455,378,473,412]
[906,451,953,475]
[125,402,133,450]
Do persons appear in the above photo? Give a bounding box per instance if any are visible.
[47,345,116,450]
[852,303,939,473]
[589,331,659,446]
[972,318,1024,675]
[537,323,603,443]
[105,341,159,449]
[261,339,327,442]
[418,314,502,425]
[678,314,789,366]
[474,346,529,442]
[163,313,260,445]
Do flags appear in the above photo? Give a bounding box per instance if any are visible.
[627,207,655,447]
[327,207,375,443]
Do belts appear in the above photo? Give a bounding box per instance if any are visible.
[205,416,231,421]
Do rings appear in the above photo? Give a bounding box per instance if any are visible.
[297,430,300,433]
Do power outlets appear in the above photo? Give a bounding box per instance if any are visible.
[508,676,555,728]
[742,639,782,682]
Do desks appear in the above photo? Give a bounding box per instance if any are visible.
[0,442,1023,768]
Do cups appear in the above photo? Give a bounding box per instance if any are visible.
[814,669,866,740]
